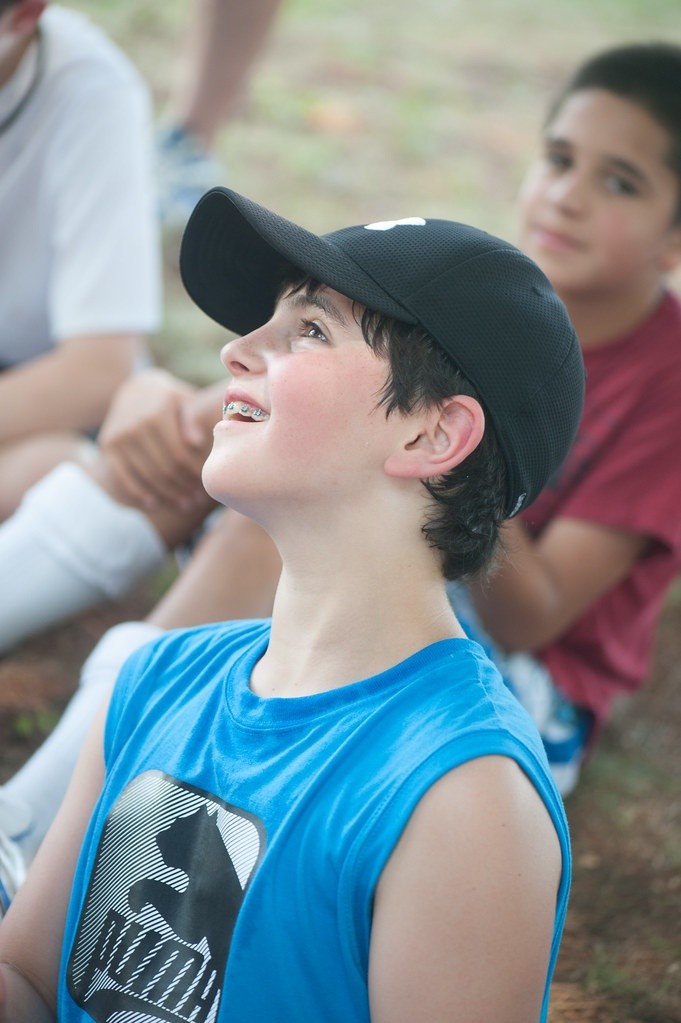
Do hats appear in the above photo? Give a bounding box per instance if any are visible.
[179,185,586,521]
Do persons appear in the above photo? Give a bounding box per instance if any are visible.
[1,0,681,926]
[0,185,585,1023]
